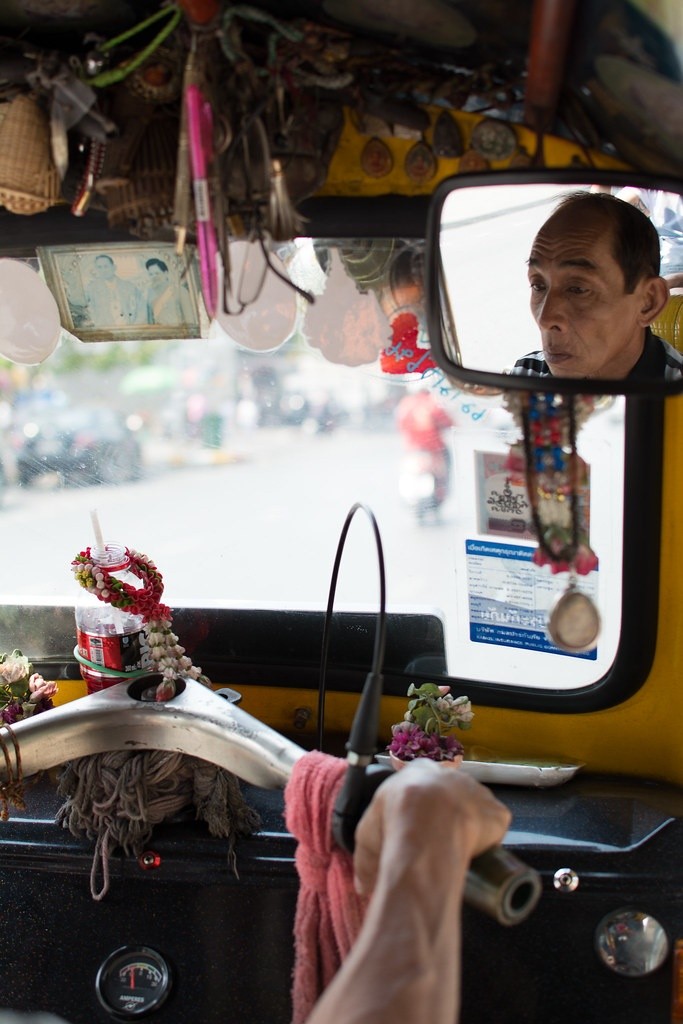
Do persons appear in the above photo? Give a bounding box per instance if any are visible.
[394,388,452,502]
[514,193,683,381]
[590,184,683,275]
[66,255,195,326]
[303,758,512,1024]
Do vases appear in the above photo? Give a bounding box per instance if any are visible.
[389,735,462,771]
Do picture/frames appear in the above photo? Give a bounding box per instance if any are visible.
[35,242,210,344]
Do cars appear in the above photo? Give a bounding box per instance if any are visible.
[12,411,144,488]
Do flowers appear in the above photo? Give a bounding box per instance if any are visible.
[0,649,57,728]
[70,546,211,701]
[385,682,474,760]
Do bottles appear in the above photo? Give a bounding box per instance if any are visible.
[75,543,155,696]
